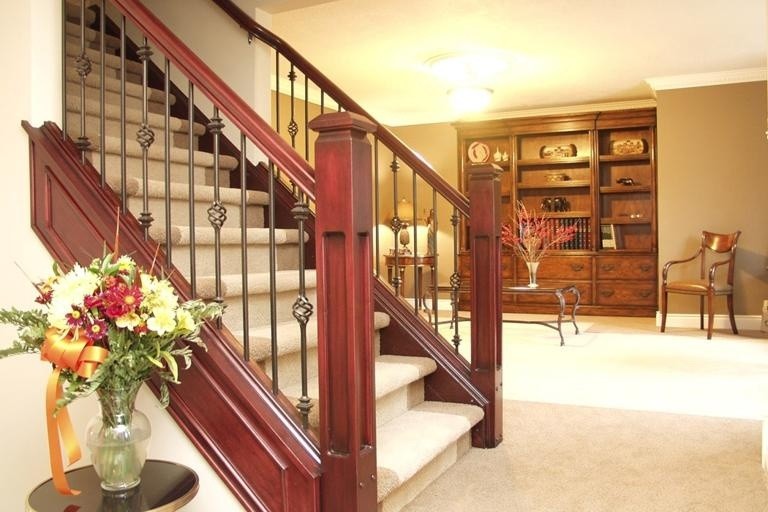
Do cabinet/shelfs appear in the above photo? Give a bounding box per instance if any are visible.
[449,107,658,317]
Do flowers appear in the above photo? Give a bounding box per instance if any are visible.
[499,199,580,262]
[0,206,225,420]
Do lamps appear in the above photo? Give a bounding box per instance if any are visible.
[396,196,415,255]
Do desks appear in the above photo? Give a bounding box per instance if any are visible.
[21,459,199,512]
[449,283,580,346]
[384,255,435,314]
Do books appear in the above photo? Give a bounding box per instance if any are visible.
[517,219,591,251]
[601,224,623,249]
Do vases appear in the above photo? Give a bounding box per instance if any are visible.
[525,262,539,288]
[84,409,153,501]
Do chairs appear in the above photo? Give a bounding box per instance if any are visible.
[660,231,741,340]
